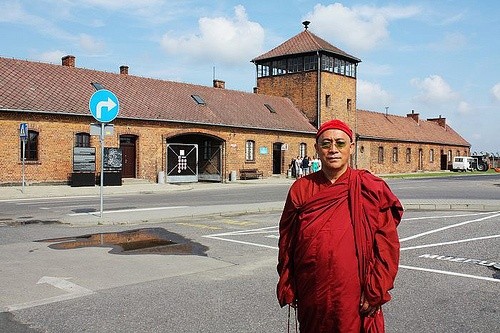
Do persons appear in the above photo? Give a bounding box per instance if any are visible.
[288,155,322,179]
[276,120,405,333]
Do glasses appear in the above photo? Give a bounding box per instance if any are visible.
[318,141,350,149]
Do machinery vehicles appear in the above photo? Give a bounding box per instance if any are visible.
[453,153,500,173]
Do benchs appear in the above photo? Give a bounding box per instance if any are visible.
[239,169,263,179]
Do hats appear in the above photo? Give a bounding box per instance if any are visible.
[316,118,353,142]
